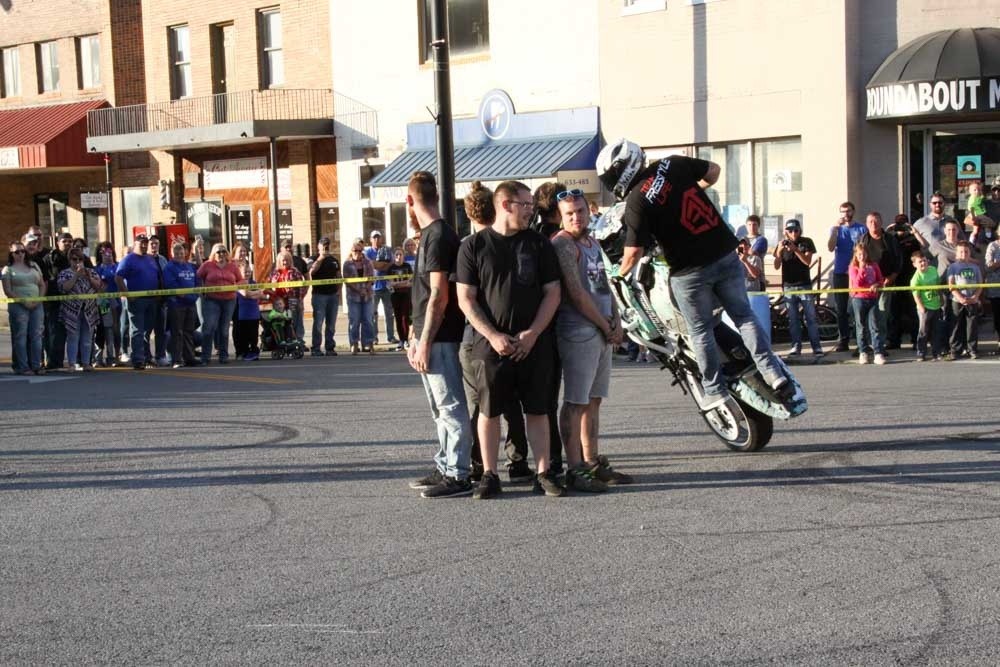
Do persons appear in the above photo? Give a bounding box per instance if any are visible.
[849,178,1000,363]
[343,230,422,356]
[828,201,869,352]
[309,237,342,356]
[456,181,570,498]
[773,220,826,356]
[406,170,475,499]
[735,215,768,292]
[0,224,309,376]
[551,192,635,491]
[462,182,573,487]
[595,138,789,411]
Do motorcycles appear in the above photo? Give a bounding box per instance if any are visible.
[589,197,809,451]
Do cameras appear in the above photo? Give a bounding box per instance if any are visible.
[894,225,912,232]
[782,239,789,246]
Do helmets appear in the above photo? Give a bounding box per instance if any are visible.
[596,136,646,201]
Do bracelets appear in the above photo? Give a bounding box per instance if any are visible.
[316,260,321,265]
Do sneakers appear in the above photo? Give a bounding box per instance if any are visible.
[409,453,637,502]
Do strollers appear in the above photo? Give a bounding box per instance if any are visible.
[258,288,305,360]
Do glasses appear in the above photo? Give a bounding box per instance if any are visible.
[931,201,944,205]
[508,199,536,211]
[353,250,362,253]
[71,257,84,262]
[12,249,26,255]
[786,230,799,233]
[556,189,587,207]
[216,250,226,253]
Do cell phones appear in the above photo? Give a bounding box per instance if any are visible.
[77,262,85,275]
[738,246,743,254]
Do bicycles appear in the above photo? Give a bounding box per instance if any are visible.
[769,296,839,340]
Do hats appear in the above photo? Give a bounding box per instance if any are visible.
[281,239,292,248]
[319,237,330,246]
[370,230,382,238]
[739,236,750,245]
[136,234,149,242]
[785,219,800,229]
[991,176,1000,190]
[21,234,39,244]
[893,214,912,232]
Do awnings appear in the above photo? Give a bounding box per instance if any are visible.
[863,26,1000,121]
[362,134,600,207]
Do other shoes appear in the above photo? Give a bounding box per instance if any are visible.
[699,391,730,411]
[10,334,413,378]
[761,363,787,392]
[610,332,1000,365]
[969,234,976,246]
